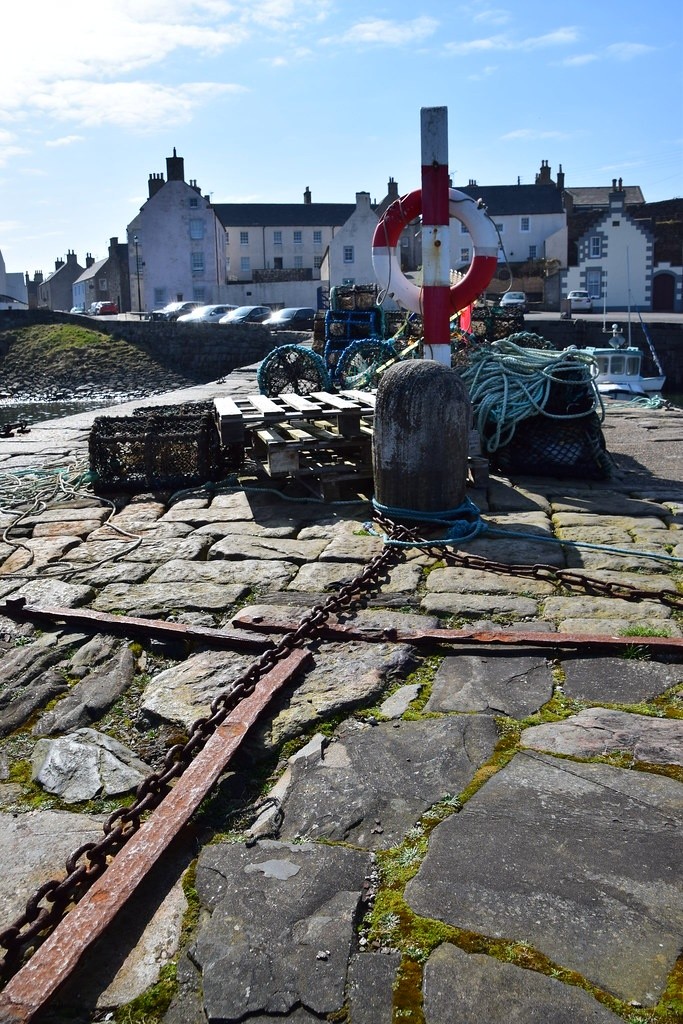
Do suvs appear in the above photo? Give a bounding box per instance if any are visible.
[152,302,204,322]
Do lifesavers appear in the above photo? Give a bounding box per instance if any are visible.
[370,186,501,320]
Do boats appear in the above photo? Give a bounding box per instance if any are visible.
[563,246,666,398]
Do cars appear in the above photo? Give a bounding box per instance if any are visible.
[177,305,238,323]
[71,307,87,314]
[219,306,271,323]
[499,292,528,313]
[262,308,316,330]
[95,301,117,316]
[567,291,592,313]
